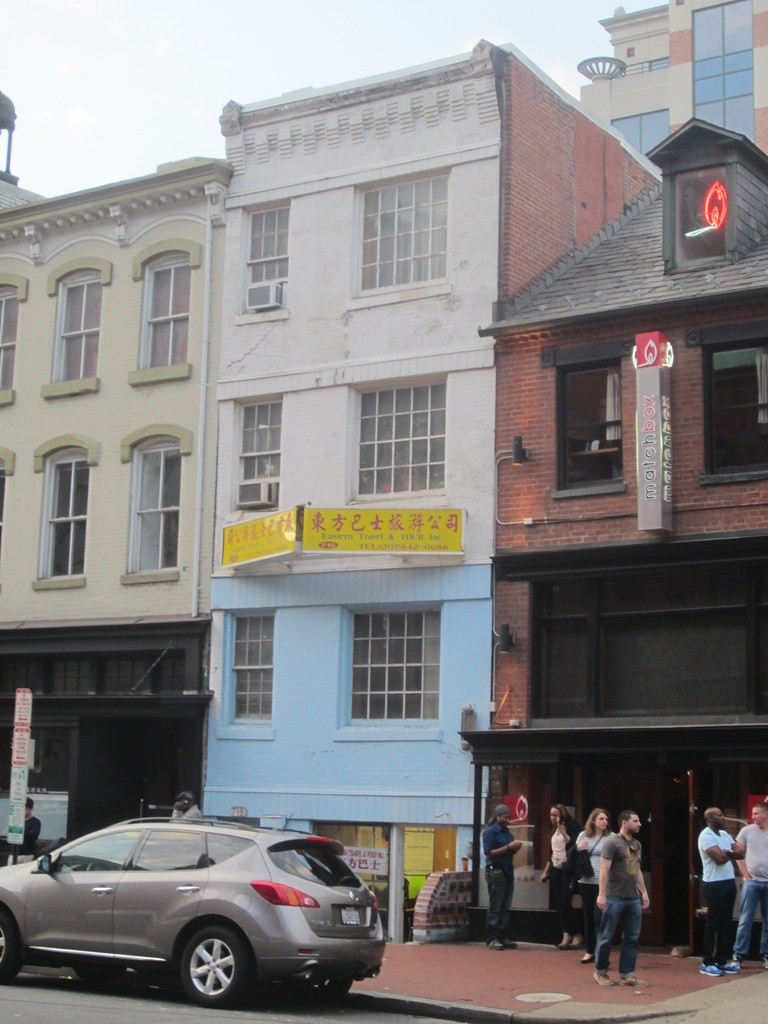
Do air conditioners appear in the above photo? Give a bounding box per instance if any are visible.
[246,283,282,310]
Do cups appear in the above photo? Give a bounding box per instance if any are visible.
[462,857,467,871]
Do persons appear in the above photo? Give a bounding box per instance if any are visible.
[367,873,430,941]
[593,810,650,988]
[540,804,587,950]
[567,808,618,964]
[697,807,740,977]
[170,792,204,826]
[729,802,768,969]
[7,798,42,872]
[482,805,522,951]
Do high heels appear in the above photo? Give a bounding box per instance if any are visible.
[558,938,571,950]
[567,939,585,950]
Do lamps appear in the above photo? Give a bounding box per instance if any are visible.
[461,705,475,750]
[512,436,525,467]
[236,481,277,507]
[499,624,512,654]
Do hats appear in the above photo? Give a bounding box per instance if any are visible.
[488,804,513,826]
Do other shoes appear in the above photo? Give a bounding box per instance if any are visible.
[593,969,615,986]
[500,936,517,950]
[580,953,595,963]
[619,975,647,986]
[488,937,504,950]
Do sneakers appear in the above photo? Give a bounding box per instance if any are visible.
[731,953,744,969]
[714,961,739,974]
[699,962,724,977]
[761,955,768,968]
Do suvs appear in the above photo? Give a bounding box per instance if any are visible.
[0,817,386,1009]
